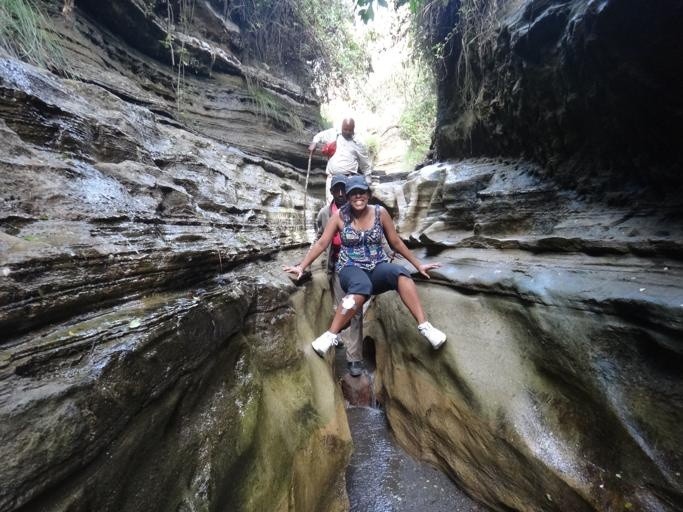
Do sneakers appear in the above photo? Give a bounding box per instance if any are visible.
[312,331,338,358]
[417,322,446,351]
[335,331,345,349]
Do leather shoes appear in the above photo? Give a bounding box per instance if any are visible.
[348,361,361,377]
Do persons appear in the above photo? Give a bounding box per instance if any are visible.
[307,176,403,377]
[281,175,446,356]
[307,116,371,205]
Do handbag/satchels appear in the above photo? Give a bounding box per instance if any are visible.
[321,134,339,157]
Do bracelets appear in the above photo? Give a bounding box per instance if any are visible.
[296,265,303,272]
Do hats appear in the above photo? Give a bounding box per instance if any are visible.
[330,174,347,189]
[344,175,369,195]
